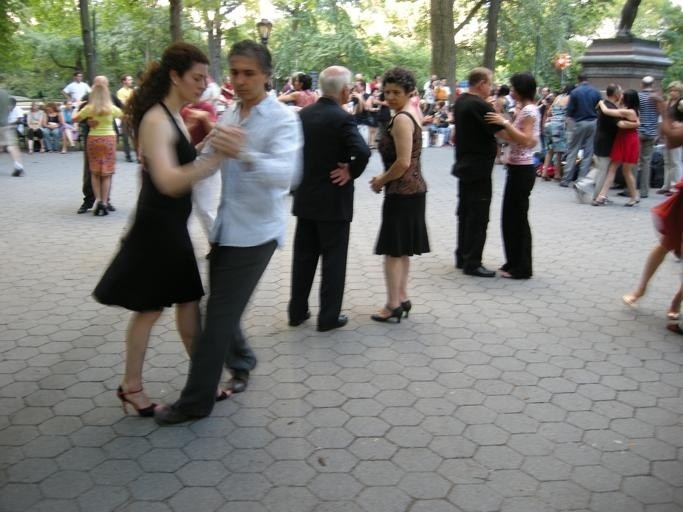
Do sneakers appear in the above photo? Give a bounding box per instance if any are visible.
[542,171,672,206]
[12,165,24,176]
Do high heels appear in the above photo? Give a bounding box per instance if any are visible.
[371,301,411,323]
[215,391,230,401]
[117,385,158,417]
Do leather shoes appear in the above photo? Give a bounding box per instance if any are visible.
[231,371,248,392]
[668,304,680,319]
[77,201,116,215]
[463,264,495,277]
[666,323,683,336]
[622,295,639,308]
[289,311,310,326]
[153,404,194,425]
[318,315,347,332]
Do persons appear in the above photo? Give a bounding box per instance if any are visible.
[1,71,149,177]
[363,65,432,325]
[494,70,683,207]
[88,41,234,418]
[621,179,683,337]
[340,73,396,150]
[180,75,239,259]
[264,72,319,112]
[296,66,373,331]
[409,74,470,148]
[482,88,495,109]
[70,78,127,217]
[77,74,127,214]
[450,66,518,279]
[150,42,306,425]
[478,71,541,280]
